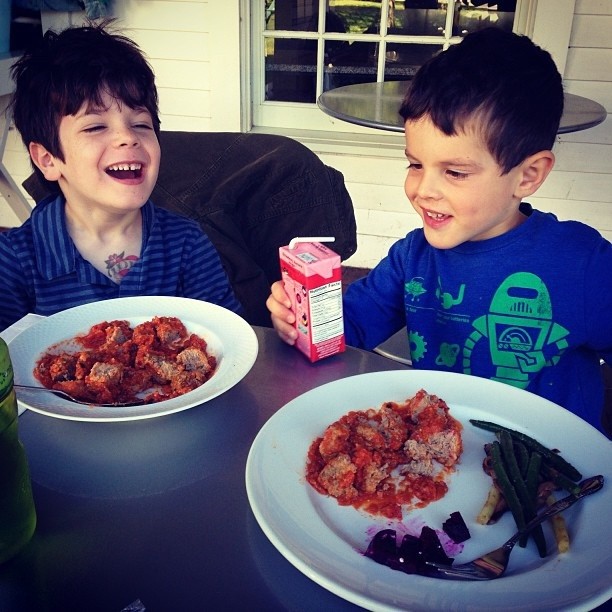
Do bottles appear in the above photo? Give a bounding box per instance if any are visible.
[0,337,37,562]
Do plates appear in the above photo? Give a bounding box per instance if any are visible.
[3,294,259,423]
[242,368,612,612]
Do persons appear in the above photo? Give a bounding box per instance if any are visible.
[265,26,612,442]
[0,17,244,331]
